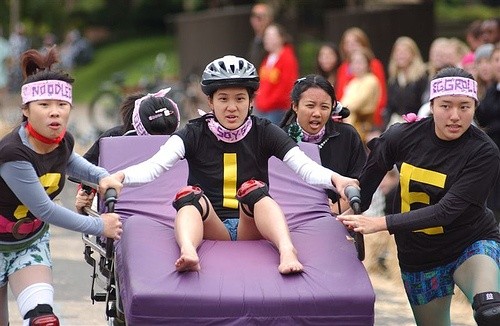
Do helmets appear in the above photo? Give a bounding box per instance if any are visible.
[200,56,260,95]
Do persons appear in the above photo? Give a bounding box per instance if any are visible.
[421,17,500,149]
[277,74,367,214]
[315,27,388,145]
[243,0,280,73]
[337,66,500,326]
[251,22,300,125]
[67,86,180,248]
[0,45,123,326]
[98,55,360,274]
[386,36,429,126]
